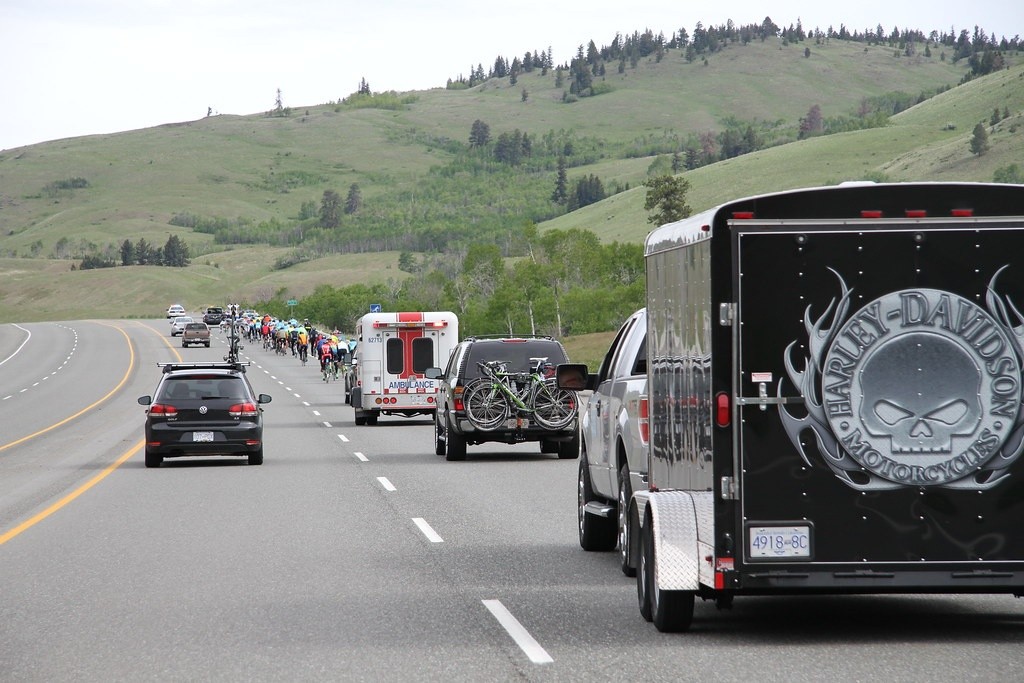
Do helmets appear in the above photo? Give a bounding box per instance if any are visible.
[322,335,326,339]
[338,337,342,342]
[350,337,357,340]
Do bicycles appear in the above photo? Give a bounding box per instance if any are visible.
[221,322,359,384]
[461,356,578,432]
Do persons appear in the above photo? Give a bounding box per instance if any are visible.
[296,332,309,363]
[219,305,357,382]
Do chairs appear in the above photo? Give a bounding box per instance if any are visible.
[172,383,189,399]
[218,381,237,398]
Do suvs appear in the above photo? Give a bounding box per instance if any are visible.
[423,333,581,462]
[554,303,648,579]
[202,307,223,325]
[138,361,272,469]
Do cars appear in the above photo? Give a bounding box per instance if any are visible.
[170,317,196,337]
[182,321,212,347]
[165,305,185,319]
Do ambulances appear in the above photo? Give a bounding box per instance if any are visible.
[351,311,459,426]
